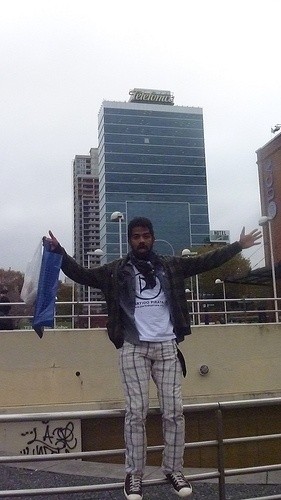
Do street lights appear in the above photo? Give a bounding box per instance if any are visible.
[182,249,200,325]
[185,288,195,325]
[110,212,124,259]
[87,249,104,328]
[258,215,278,322]
[215,278,228,325]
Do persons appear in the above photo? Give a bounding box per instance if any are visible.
[42,215,263,500]
